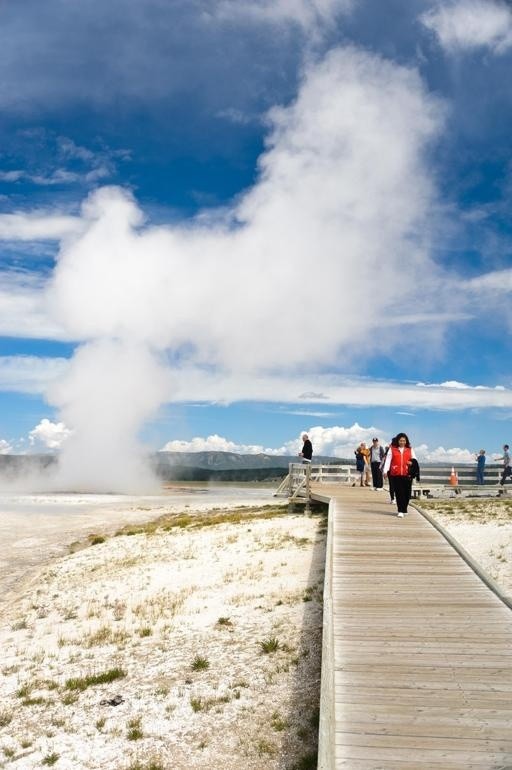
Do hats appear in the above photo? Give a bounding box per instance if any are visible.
[372,437,378,441]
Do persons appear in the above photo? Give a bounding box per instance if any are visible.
[474,449,486,485]
[378,438,396,504]
[352,447,366,487]
[367,438,385,490]
[298,434,313,488]
[360,442,370,487]
[494,444,512,497]
[382,432,418,518]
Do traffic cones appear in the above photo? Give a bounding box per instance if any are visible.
[449,465,458,486]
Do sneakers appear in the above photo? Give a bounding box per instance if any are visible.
[390,500,408,518]
